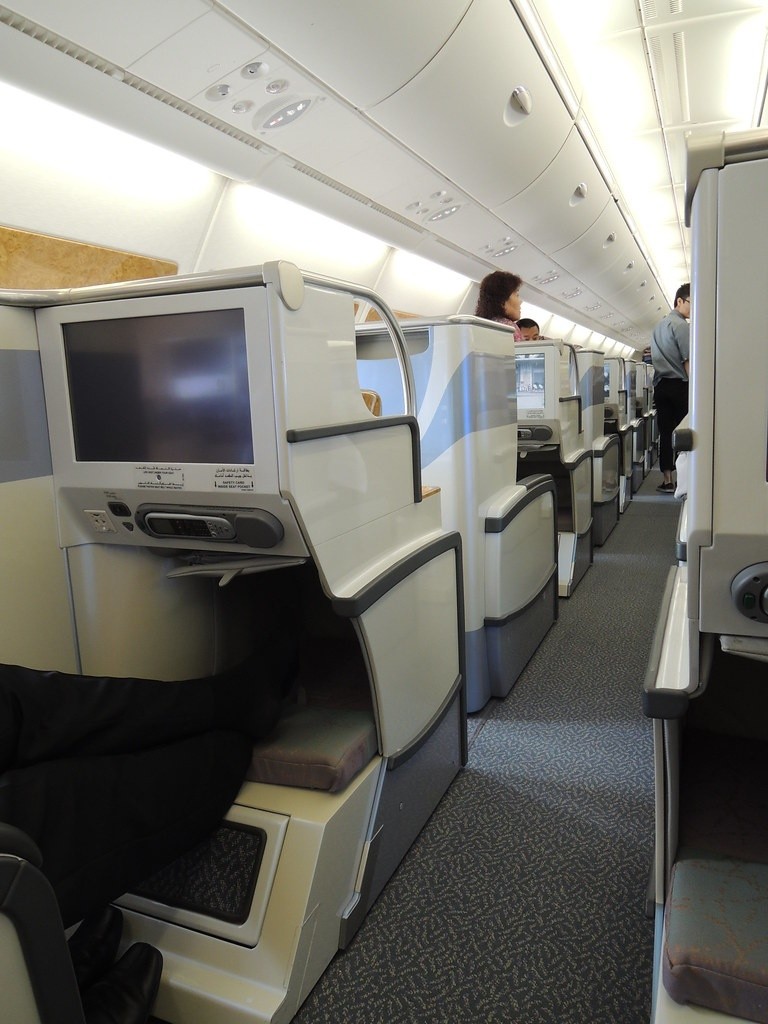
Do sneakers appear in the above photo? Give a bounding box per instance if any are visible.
[656,481,676,492]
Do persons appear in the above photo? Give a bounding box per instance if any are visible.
[0,637,295,928]
[516,318,539,341]
[651,283,691,492]
[475,271,523,342]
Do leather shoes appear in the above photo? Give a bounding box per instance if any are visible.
[76,942,164,1024]
[65,904,126,992]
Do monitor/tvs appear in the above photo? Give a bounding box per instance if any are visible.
[514,353,545,409]
[603,363,610,396]
[60,308,255,464]
[351,324,434,426]
